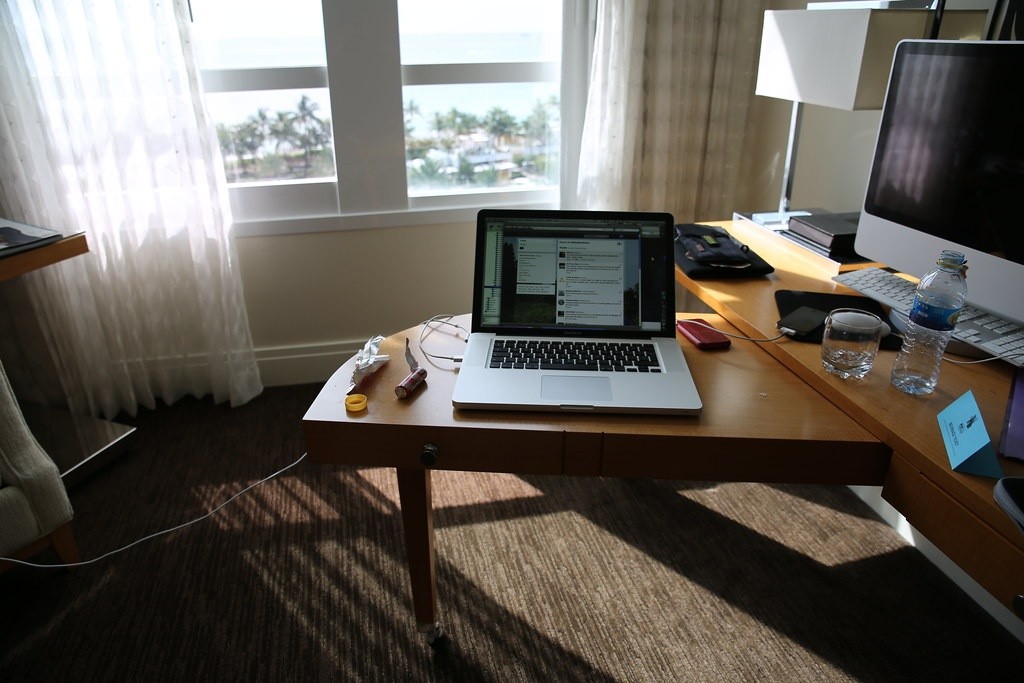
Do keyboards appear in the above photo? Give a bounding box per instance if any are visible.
[831,265,1024,367]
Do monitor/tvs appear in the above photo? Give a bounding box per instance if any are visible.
[855,40,1024,363]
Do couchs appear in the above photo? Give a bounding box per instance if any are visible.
[0,354,84,578]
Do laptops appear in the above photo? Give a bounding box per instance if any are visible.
[451,209,704,416]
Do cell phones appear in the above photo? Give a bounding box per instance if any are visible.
[994,476,1024,527]
[776,306,828,336]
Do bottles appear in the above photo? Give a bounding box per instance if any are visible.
[890,250,968,394]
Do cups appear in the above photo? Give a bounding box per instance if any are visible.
[822,308,882,379]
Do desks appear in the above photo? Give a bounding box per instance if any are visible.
[300,211,1023,649]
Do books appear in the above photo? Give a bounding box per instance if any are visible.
[780,211,873,264]
